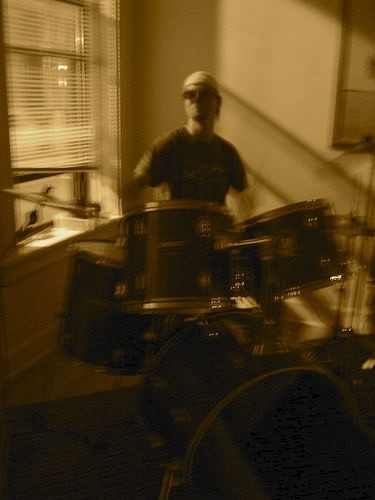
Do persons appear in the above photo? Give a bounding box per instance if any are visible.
[118,71,255,216]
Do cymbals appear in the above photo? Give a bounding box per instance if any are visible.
[2,188,104,216]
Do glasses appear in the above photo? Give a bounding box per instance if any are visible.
[184,89,213,99]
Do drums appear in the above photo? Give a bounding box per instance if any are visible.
[119,198,238,310]
[52,240,158,375]
[137,310,365,499]
[230,197,349,304]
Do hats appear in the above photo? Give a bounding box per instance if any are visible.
[184,70,219,91]
